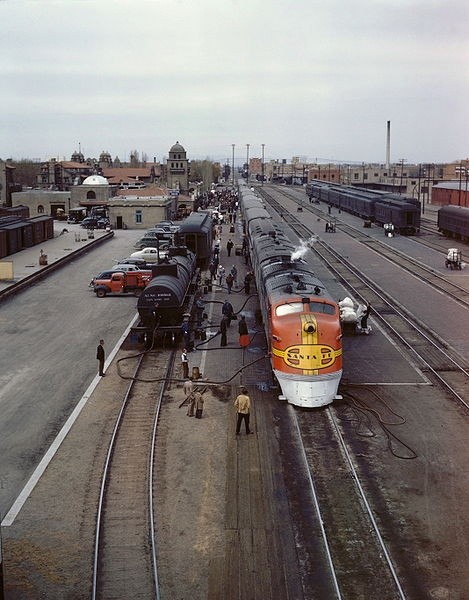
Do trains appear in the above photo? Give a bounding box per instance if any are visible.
[437,204,469,245]
[304,179,422,235]
[130,211,216,350]
[238,178,346,410]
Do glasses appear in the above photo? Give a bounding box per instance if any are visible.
[185,321,188,322]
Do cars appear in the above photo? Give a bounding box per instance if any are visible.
[135,237,170,250]
[118,259,147,269]
[67,205,109,229]
[88,271,115,289]
[110,264,139,272]
[145,220,180,239]
[132,247,169,263]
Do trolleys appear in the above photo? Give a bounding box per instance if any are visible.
[341,320,357,335]
[325,220,336,233]
[383,225,395,237]
[444,250,462,271]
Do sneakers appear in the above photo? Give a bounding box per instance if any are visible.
[246,430,254,434]
[235,432,239,435]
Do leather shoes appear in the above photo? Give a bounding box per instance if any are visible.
[189,414,195,417]
[186,413,188,416]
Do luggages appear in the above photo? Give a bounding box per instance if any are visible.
[192,367,199,379]
[239,334,250,347]
[187,340,194,352]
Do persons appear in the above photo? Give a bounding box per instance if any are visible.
[196,296,205,326]
[309,197,312,203]
[361,303,371,327]
[209,260,216,280]
[226,273,234,293]
[96,339,107,377]
[222,300,234,327]
[183,377,196,418]
[215,238,221,252]
[216,227,220,237]
[181,348,190,379]
[194,188,239,223]
[230,264,238,288]
[234,388,254,435]
[220,316,228,346]
[226,238,234,257]
[239,315,248,346]
[217,263,226,285]
[244,272,252,293]
[229,223,235,237]
[194,390,204,418]
[218,224,222,233]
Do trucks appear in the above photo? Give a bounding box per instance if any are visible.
[90,270,153,298]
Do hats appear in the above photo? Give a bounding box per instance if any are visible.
[241,389,249,393]
[183,319,188,322]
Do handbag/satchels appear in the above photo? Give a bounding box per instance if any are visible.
[203,313,208,318]
